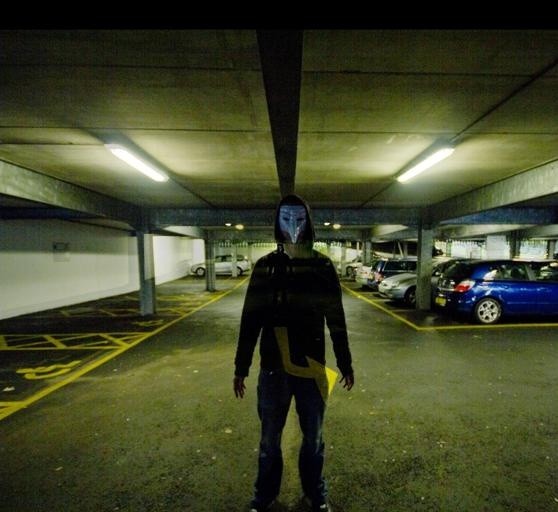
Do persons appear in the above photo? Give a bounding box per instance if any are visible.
[232,192,354,512]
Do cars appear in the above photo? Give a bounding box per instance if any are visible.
[338,252,558,325]
[191,254,252,277]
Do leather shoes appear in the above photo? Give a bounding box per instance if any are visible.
[249,497,276,512]
[301,494,328,512]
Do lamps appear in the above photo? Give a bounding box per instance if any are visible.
[102,134,171,183]
[394,139,455,183]
